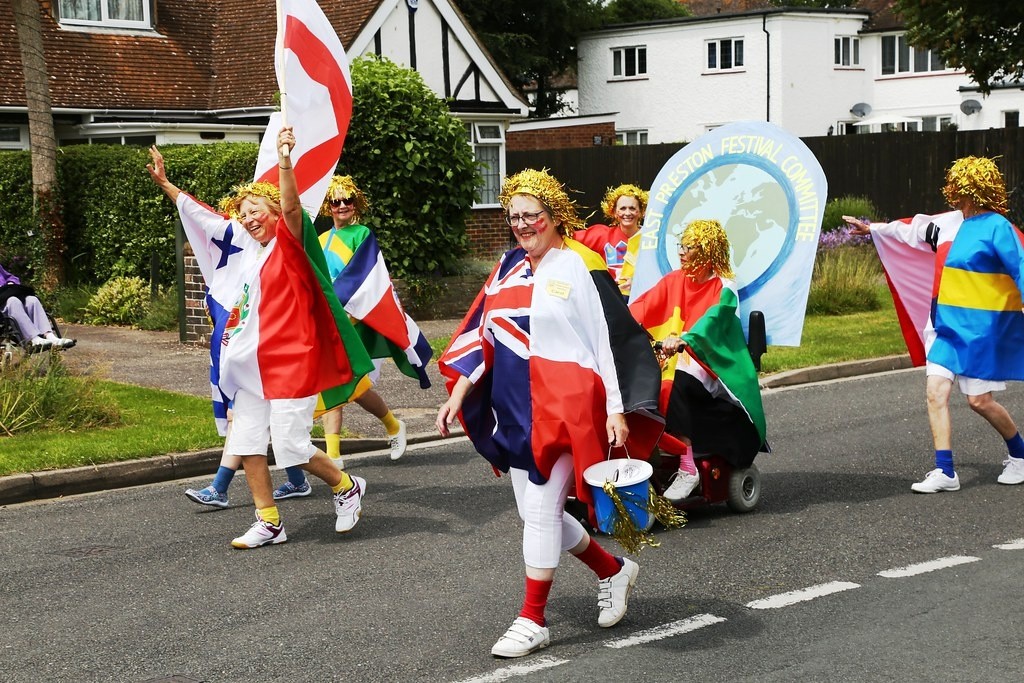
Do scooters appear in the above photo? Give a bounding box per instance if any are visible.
[636,310,770,516]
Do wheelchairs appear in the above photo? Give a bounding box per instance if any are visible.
[0,295,67,372]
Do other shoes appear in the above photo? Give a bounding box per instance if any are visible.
[30,340,52,353]
[53,338,75,349]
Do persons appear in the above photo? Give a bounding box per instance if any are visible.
[0,264,73,347]
[181,175,407,508]
[626,220,741,500]
[147,126,366,548]
[570,180,649,299]
[435,169,641,656]
[839,158,1024,491]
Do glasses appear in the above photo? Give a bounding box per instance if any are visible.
[505,208,546,227]
[324,196,356,207]
[677,243,701,254]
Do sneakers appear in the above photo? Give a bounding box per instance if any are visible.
[231,509,287,548]
[911,468,960,493]
[597,555,639,626]
[185,486,229,508]
[998,454,1024,484]
[333,475,366,531]
[663,467,700,500]
[492,617,549,657]
[273,477,312,499]
[329,457,345,469]
[387,420,406,460]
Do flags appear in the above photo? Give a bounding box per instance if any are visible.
[250,1,353,225]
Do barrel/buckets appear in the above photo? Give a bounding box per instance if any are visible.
[582,441,653,534]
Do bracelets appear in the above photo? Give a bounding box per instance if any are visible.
[279,163,292,170]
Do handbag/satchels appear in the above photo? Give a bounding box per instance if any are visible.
[0,281,35,310]
